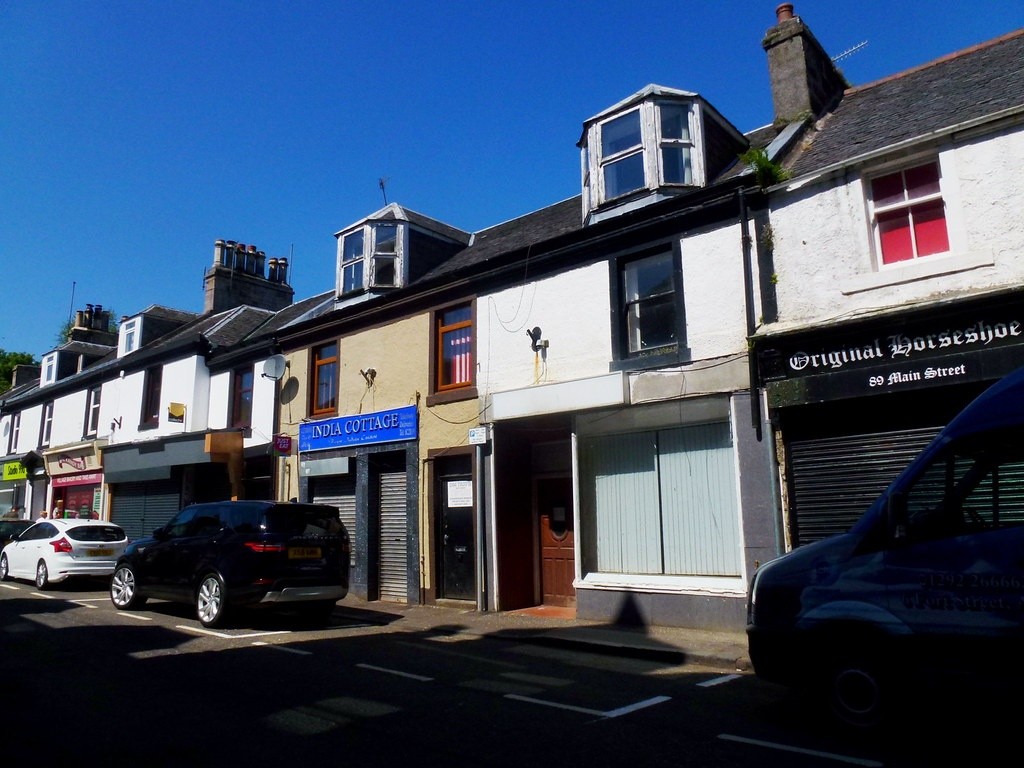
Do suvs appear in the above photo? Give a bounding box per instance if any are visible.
[109,499,353,629]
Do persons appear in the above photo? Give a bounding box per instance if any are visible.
[2,505,20,518]
[36,511,47,522]
[53,501,63,518]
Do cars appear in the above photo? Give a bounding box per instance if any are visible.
[1,515,133,590]
[745,364,1024,741]
